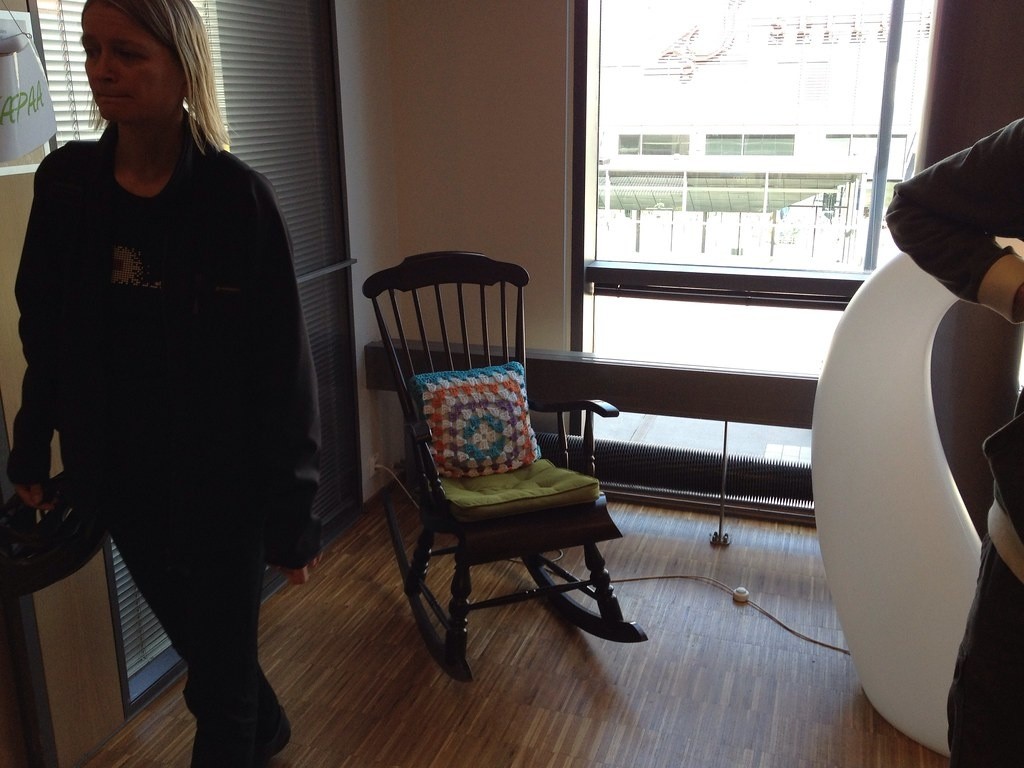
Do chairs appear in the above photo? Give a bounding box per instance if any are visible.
[361,250,648,679]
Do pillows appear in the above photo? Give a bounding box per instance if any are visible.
[407,360,542,478]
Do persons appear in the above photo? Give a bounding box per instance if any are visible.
[0,0,328,768]
[887,116,1024,768]
